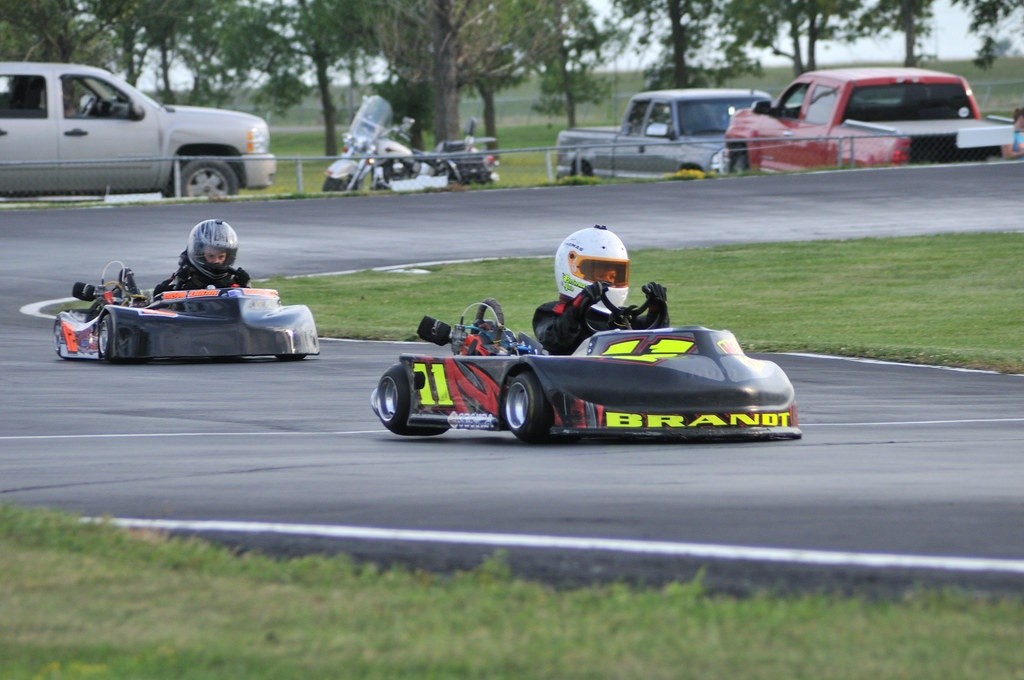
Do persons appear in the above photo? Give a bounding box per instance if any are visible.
[1000,108,1024,160]
[154,220,251,302]
[533,225,670,355]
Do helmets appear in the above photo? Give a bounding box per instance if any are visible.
[186,218,238,280]
[554,224,631,315]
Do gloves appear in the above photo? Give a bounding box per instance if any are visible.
[642,282,667,313]
[153,278,176,296]
[572,280,611,313]
[233,267,251,287]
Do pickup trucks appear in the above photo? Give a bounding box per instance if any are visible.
[0,62,274,201]
[724,65,1009,170]
[555,89,770,180]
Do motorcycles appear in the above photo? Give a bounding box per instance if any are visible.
[321,95,501,193]
